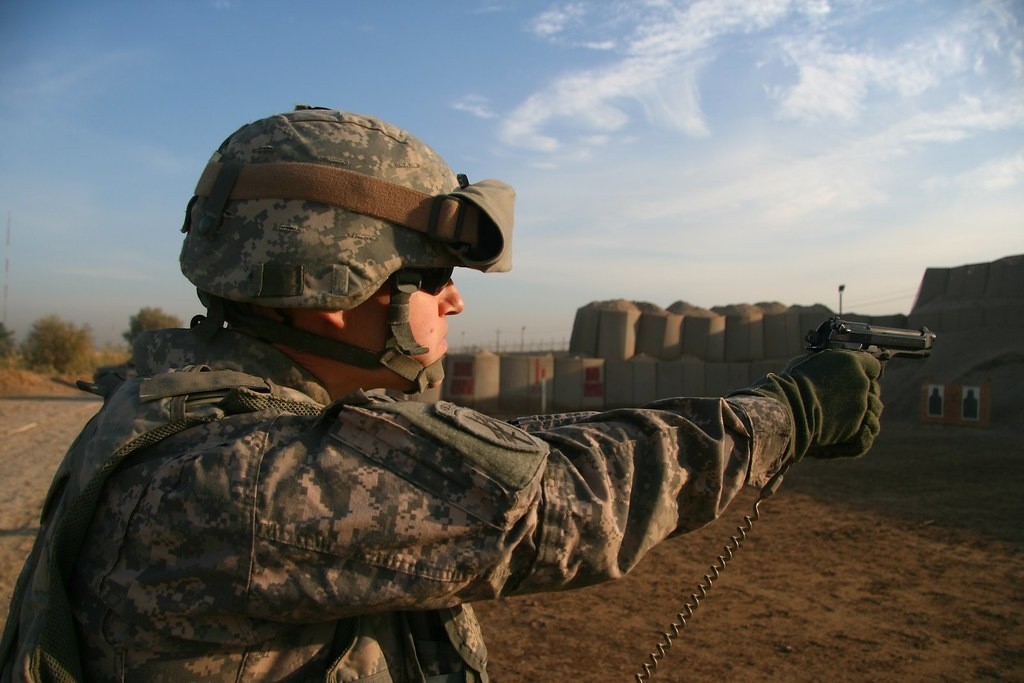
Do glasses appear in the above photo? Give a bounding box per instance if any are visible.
[389,267,454,297]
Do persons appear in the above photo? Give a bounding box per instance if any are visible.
[3,102,883,683]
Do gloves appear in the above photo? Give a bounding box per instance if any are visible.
[728,346,883,459]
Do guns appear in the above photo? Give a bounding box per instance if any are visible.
[805,319,935,379]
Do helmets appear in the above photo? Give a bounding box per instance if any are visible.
[177,104,516,309]
